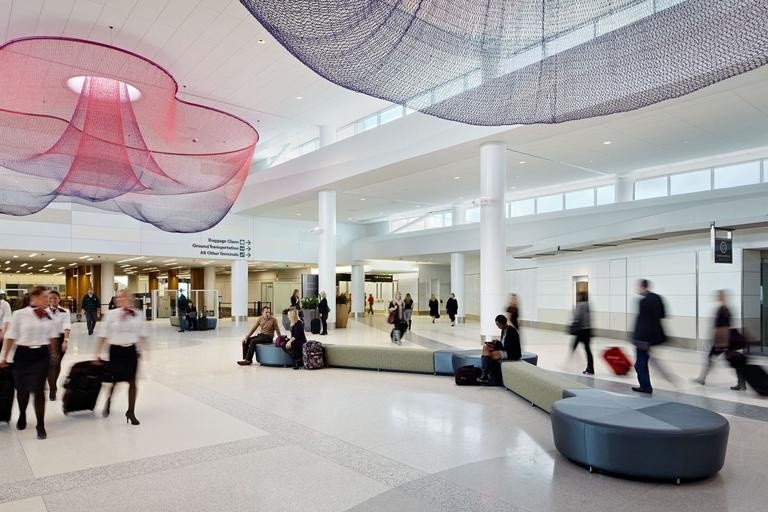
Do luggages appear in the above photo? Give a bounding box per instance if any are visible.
[309,318,321,335]
[62,361,104,416]
[390,321,409,341]
[302,340,324,369]
[0,362,14,424]
[738,361,768,397]
[603,345,632,377]
[197,316,209,332]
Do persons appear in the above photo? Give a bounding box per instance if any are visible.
[0,286,147,439]
[446,293,458,326]
[565,291,594,375]
[290,289,305,328]
[368,293,374,315]
[403,293,414,330]
[477,293,522,387]
[237,306,281,365]
[690,290,749,390]
[317,290,330,335]
[282,309,307,370]
[627,278,685,393]
[429,294,438,324]
[177,288,187,332]
[388,291,405,346]
[186,300,198,331]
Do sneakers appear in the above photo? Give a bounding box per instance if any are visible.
[731,383,747,391]
[476,376,491,383]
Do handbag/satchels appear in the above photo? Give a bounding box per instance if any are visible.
[569,319,586,337]
[728,326,750,368]
[455,363,481,386]
[274,335,288,349]
[386,312,396,326]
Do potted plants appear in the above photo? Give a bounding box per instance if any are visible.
[336,289,351,328]
[301,297,320,332]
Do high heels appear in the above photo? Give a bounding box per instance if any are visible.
[124,410,139,426]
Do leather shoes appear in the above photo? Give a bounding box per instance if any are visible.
[582,367,595,375]
[237,360,252,365]
[50,385,58,401]
[630,385,653,394]
[16,414,28,430]
[293,358,303,371]
[35,423,48,440]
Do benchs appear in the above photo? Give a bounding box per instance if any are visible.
[501,359,729,483]
[169,315,217,331]
[252,332,538,387]
